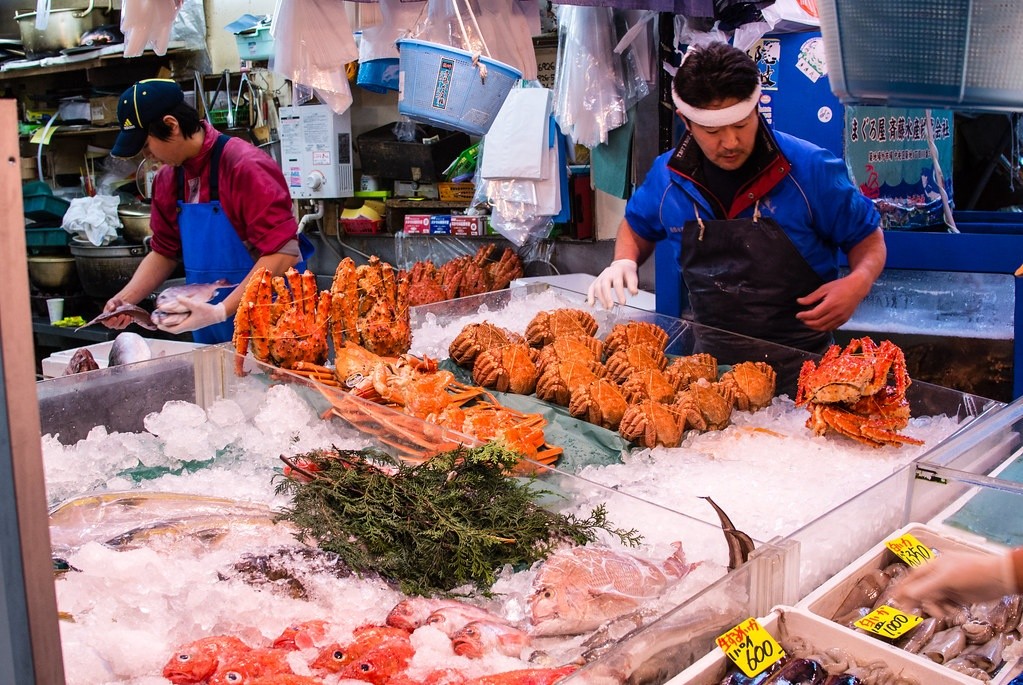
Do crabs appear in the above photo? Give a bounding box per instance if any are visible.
[232,243,925,479]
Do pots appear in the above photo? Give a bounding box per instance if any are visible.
[28,156,166,298]
[13,0,114,61]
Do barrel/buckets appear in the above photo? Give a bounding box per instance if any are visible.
[235,27,272,61]
[396,37,522,138]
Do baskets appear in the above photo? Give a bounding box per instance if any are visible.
[395,37,523,138]
[816,1,1023,114]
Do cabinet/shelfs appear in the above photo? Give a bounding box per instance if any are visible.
[0,37,211,141]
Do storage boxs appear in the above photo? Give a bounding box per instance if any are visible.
[31,263,1022,684]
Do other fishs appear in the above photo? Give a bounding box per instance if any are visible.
[150,277,241,326]
[74,304,157,332]
[47,448,394,573]
[162,494,757,685]
[719,608,920,685]
[36,347,101,383]
[831,547,1023,682]
[107,331,152,376]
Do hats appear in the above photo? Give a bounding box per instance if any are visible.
[110,77,185,159]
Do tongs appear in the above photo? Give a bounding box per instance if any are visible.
[192,71,212,124]
[234,73,256,127]
[203,69,233,127]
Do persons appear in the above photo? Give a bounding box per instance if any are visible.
[587,41,887,405]
[891,546,1023,620]
[99,78,317,347]
